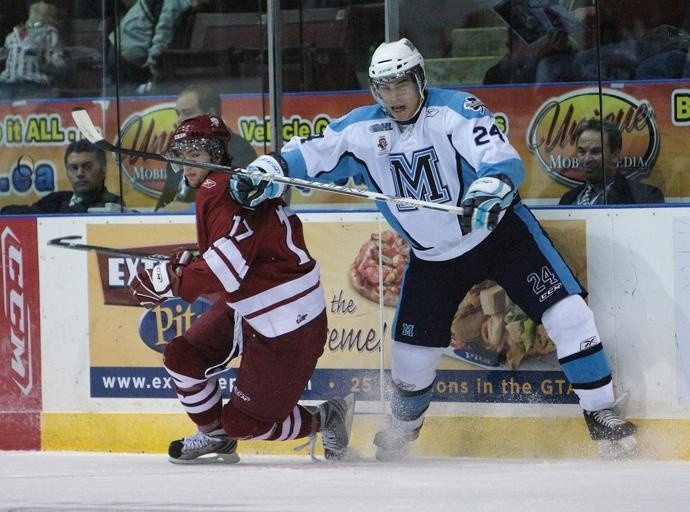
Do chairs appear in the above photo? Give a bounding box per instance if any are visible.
[0,0,386,100]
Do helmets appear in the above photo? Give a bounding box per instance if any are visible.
[366,37,426,113]
[169,114,230,159]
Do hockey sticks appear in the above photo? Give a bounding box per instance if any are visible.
[48,235,154,261]
[69,105,465,217]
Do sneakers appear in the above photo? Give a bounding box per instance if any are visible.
[373,425,420,450]
[582,407,636,442]
[316,396,348,462]
[167,425,239,461]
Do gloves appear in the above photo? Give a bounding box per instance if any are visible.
[226,151,290,210]
[456,176,514,237]
[126,249,192,310]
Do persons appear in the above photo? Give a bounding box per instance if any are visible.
[132,115,348,460]
[229,37,637,451]
